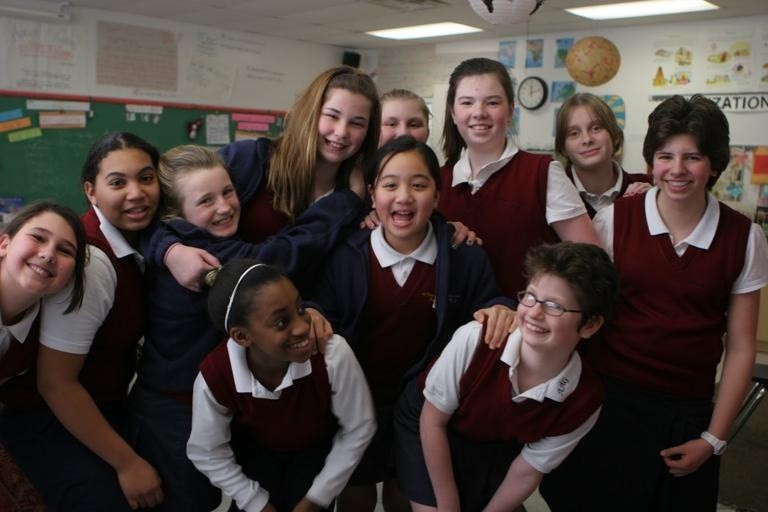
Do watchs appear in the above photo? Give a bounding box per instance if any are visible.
[700,432,729,457]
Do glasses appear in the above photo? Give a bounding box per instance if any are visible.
[516,290,589,318]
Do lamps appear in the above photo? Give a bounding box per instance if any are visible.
[470,0,539,26]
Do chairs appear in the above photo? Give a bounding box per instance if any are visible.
[727,364,768,443]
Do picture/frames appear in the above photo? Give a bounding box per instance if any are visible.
[519,78,548,110]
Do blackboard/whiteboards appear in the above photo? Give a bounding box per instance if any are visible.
[0,90,289,231]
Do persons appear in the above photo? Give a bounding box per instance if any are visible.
[440,58,603,295]
[592,95,766,507]
[128,68,381,512]
[306,135,520,507]
[187,258,377,507]
[36,132,165,512]
[0,204,88,509]
[554,92,654,219]
[145,146,366,505]
[399,242,621,512]
[361,90,484,249]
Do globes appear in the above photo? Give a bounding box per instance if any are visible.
[566,36,620,86]
